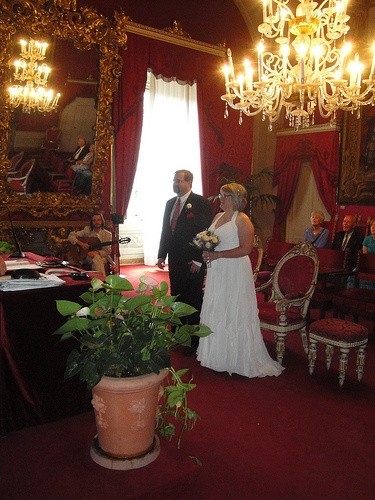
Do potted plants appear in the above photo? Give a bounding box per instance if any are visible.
[53,275,215,472]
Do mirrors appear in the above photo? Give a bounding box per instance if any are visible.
[0,1,128,220]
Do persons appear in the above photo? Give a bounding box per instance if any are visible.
[358,219,375,290]
[67,137,89,166]
[194,183,287,378]
[68,212,112,274]
[332,215,365,275]
[157,169,213,356]
[303,209,330,248]
[71,144,94,196]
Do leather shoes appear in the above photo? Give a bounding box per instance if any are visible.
[183,342,197,355]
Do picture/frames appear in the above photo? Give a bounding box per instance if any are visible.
[334,106,375,210]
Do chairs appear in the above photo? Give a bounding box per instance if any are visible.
[332,249,375,327]
[4,144,85,194]
[308,247,346,320]
[255,241,317,373]
[254,240,294,299]
[321,215,374,263]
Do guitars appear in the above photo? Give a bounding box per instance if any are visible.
[72,236,131,261]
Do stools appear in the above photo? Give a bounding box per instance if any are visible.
[307,316,368,387]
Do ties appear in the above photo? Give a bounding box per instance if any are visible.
[171,199,181,236]
[341,233,348,250]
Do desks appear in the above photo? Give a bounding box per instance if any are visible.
[0,255,110,436]
[0,225,115,271]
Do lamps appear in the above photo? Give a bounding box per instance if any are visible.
[216,0,374,131]
[7,33,63,116]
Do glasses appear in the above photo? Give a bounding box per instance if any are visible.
[218,193,232,199]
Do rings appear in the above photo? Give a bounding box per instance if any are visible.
[207,256,210,260]
[207,260,210,263]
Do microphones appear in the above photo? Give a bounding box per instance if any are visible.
[6,206,26,258]
